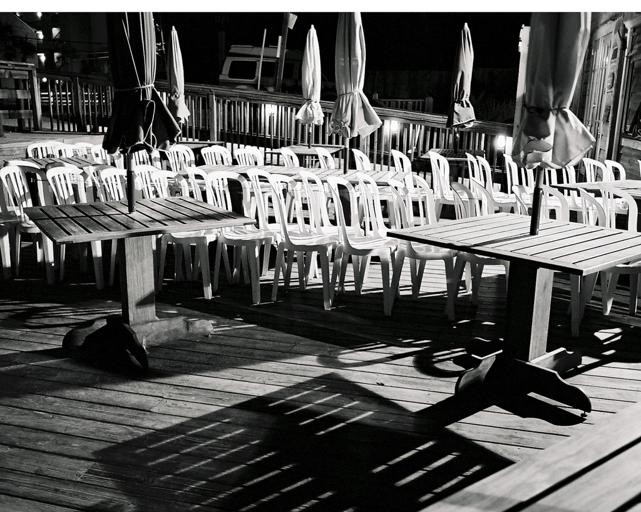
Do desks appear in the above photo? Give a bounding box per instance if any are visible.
[22,199,253,377]
[387,212,641,414]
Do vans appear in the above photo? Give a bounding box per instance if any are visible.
[219,44,337,94]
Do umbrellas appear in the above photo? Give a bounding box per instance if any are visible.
[101,12,181,213]
[164,24,191,133]
[294,23,326,146]
[445,20,478,152]
[329,11,385,174]
[510,12,598,234]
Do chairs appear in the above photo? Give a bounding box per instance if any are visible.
[1,137,638,322]
